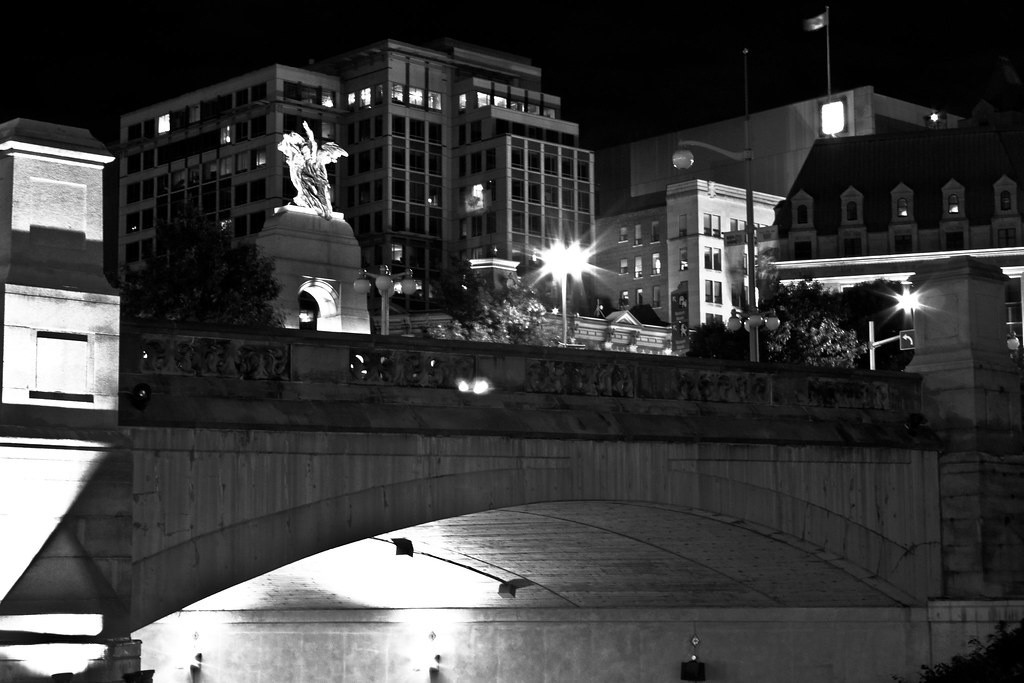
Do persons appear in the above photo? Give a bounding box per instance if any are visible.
[277,120,350,220]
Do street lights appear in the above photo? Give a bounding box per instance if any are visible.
[544,242,591,349]
[672,47,781,364]
[353,264,417,379]
